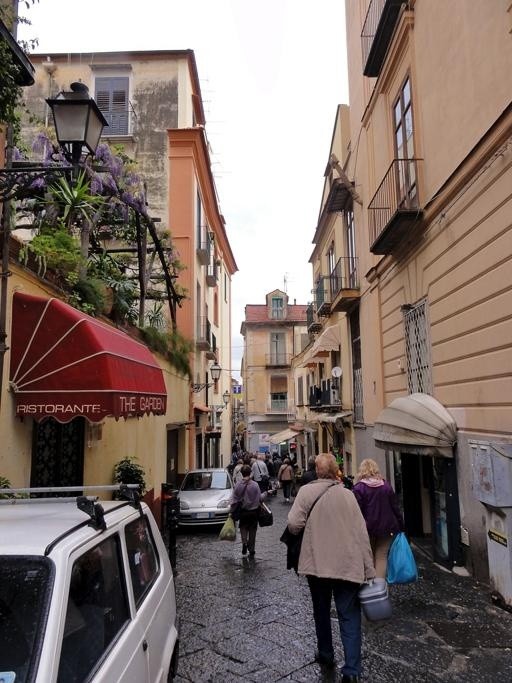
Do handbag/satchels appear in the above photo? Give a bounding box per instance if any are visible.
[262,475,269,486]
[230,503,241,520]
[259,502,273,526]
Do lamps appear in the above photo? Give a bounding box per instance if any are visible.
[192,359,222,392]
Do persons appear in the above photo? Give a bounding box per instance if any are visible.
[350,457,406,582]
[228,464,265,562]
[285,452,376,682]
[231,445,298,504]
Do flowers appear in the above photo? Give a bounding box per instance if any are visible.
[12,130,185,311]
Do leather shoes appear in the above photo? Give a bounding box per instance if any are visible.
[242,543,247,554]
[342,675,360,683]
[315,653,334,667]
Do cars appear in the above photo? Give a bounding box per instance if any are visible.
[177,467,236,526]
[0,483,183,682]
